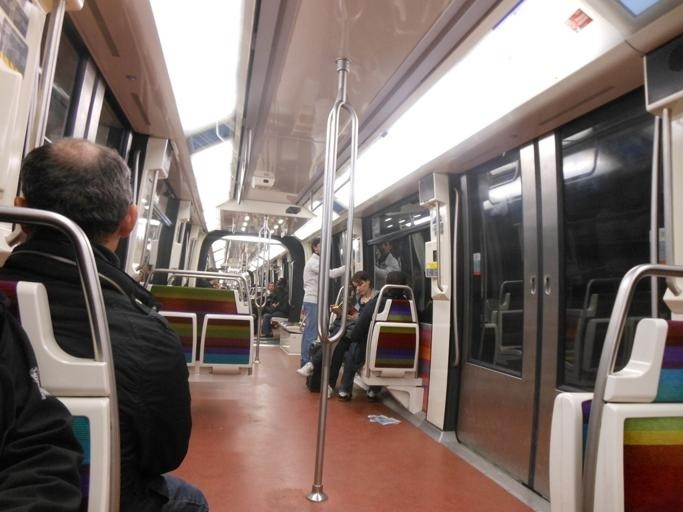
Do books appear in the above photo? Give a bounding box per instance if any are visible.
[330,301,359,320]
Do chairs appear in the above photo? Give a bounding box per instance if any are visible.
[328,285,345,338]
[0,204,122,512]
[357,285,425,418]
[136,268,259,384]
[549,263,683,512]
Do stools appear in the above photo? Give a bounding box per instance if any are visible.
[270,316,288,339]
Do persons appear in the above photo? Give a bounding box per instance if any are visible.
[373,238,401,285]
[337,271,409,401]
[300,238,346,375]
[328,271,381,402]
[196,267,220,290]
[296,288,362,392]
[259,278,289,337]
[1,139,211,512]
[1,292,86,512]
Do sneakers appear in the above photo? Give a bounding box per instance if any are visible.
[367,390,376,401]
[296,362,314,376]
[338,392,350,401]
[327,385,332,398]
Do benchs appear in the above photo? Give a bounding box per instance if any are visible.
[279,321,309,355]
